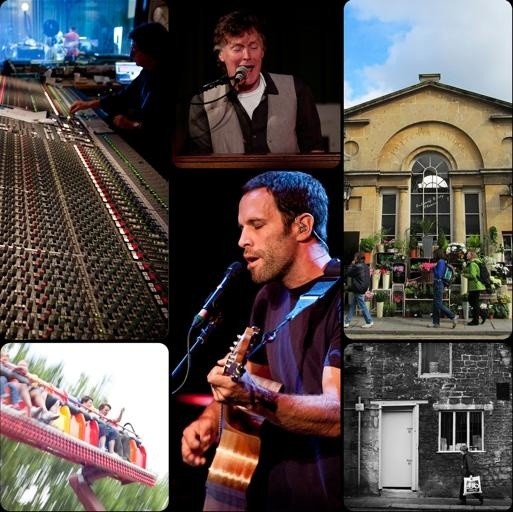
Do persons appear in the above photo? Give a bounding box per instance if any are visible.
[0,351,127,457]
[427,249,460,329]
[63,27,79,60]
[463,249,487,325]
[343,252,373,328]
[188,12,327,155]
[458,446,484,504]
[180,170,342,512]
[69,23,170,182]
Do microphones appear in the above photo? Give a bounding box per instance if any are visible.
[234,66,248,84]
[191,261,243,329]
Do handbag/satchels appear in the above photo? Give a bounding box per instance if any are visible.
[463,475,483,496]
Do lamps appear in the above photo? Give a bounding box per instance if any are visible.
[342,179,353,210]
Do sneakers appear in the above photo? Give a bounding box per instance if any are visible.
[29,407,59,420]
[452,314,459,328]
[428,323,440,328]
[344,322,373,328]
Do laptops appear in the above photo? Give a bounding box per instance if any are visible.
[115,62,143,85]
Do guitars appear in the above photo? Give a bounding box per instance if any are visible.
[202,328,285,512]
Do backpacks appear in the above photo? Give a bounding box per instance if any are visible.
[472,260,490,284]
[444,264,458,288]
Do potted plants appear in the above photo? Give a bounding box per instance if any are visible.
[359,221,509,318]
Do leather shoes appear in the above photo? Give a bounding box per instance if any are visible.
[468,309,488,325]
[456,500,466,505]
[479,496,484,505]
